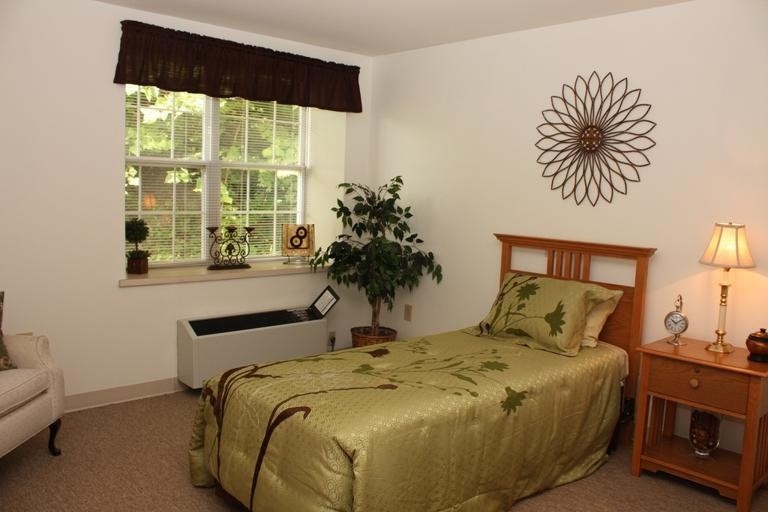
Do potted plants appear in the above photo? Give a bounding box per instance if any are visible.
[307,171,444,351]
[124,216,157,276]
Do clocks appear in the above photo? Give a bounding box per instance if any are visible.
[661,294,688,348]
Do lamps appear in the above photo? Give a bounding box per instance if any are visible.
[697,220,757,356]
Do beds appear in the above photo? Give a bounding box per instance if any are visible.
[182,230,661,511]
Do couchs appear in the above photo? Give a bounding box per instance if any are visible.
[0,331,68,463]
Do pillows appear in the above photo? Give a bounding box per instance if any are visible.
[0,290,19,371]
[461,270,615,358]
[575,286,625,348]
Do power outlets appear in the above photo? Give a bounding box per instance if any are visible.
[327,329,337,347]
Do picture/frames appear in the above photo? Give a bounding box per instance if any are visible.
[280,222,316,257]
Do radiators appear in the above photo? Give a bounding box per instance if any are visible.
[175,303,328,394]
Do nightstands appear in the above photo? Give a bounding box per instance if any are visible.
[628,334,768,511]
